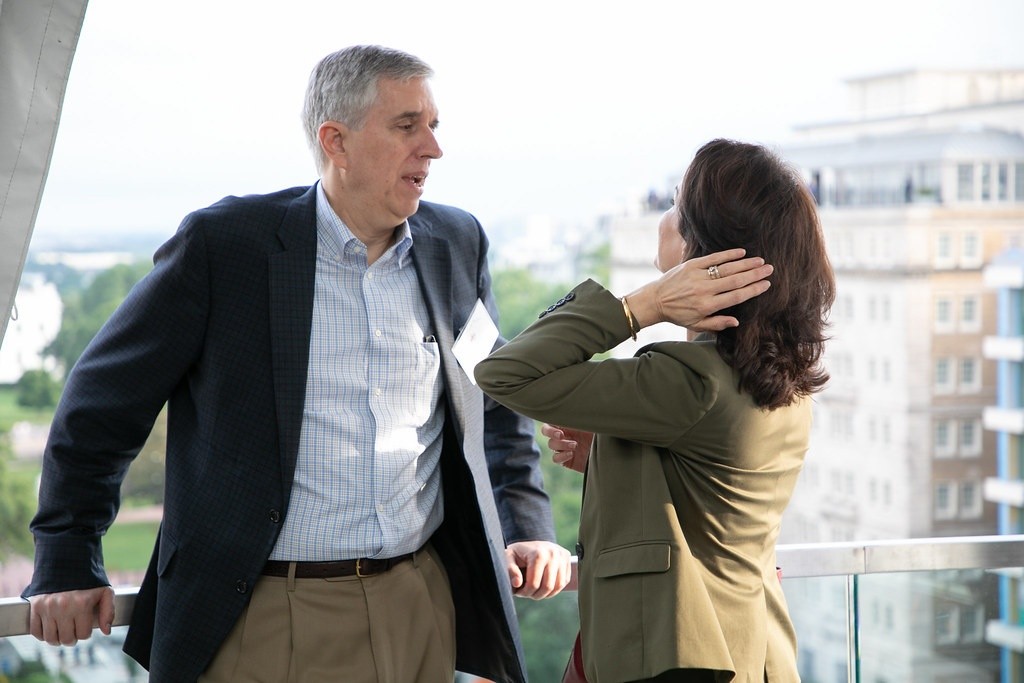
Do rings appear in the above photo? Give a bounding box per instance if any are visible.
[708,265,719,280]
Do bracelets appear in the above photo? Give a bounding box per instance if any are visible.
[621,296,638,341]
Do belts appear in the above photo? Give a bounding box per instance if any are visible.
[263,544,428,579]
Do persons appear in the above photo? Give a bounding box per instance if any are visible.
[473,136,838,682]
[18,45,574,683]
